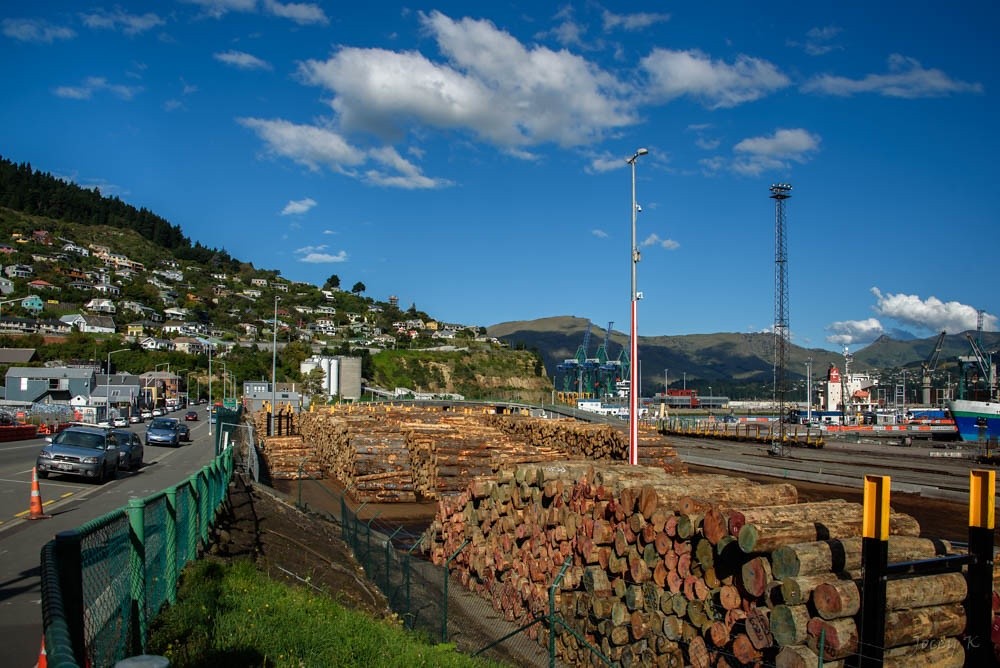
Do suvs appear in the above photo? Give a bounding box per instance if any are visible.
[36,423,120,485]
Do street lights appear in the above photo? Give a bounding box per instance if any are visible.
[626,146,649,465]
[194,337,212,436]
[154,363,172,408]
[106,348,132,421]
[208,358,237,400]
[176,369,196,408]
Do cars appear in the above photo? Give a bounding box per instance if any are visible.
[185,411,198,421]
[152,399,204,417]
[108,429,144,470]
[113,417,130,427]
[205,402,222,424]
[99,419,115,426]
[129,414,145,424]
[178,424,191,442]
[141,410,154,420]
[145,418,181,449]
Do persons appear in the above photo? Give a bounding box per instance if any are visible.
[722,407,735,423]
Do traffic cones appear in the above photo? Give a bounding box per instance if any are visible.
[23,466,52,519]
